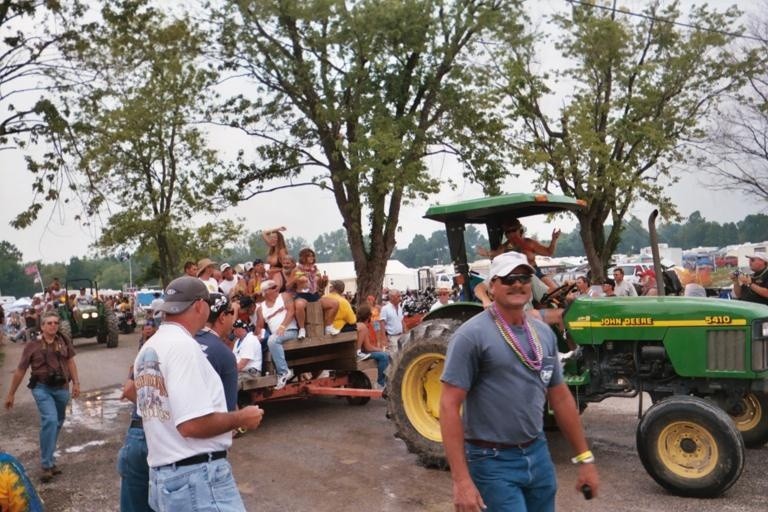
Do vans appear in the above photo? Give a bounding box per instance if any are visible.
[606,262,655,297]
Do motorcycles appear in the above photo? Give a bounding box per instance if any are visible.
[119,310,137,333]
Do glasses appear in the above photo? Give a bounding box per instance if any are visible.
[506,227,519,234]
[269,284,278,289]
[494,273,534,285]
[225,309,234,315]
[46,321,59,325]
[439,293,446,296]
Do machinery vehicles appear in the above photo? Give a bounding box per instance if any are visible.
[382,192,767,497]
[59,278,119,348]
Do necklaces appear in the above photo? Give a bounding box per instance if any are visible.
[488,301,544,372]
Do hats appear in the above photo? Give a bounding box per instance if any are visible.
[21,285,86,316]
[489,251,537,281]
[152,277,211,315]
[599,278,615,286]
[434,284,454,295]
[745,253,768,263]
[638,270,656,278]
[196,255,297,277]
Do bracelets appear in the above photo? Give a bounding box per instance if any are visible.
[571,451,595,465]
[747,282,753,288]
[72,382,80,385]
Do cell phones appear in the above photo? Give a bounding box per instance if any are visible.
[582,487,591,500]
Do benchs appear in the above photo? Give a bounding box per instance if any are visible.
[239,302,360,391]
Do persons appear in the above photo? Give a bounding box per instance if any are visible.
[437,251,599,512]
[728,252,768,305]
[134,275,265,512]
[428,217,659,313]
[0,277,131,343]
[117,292,239,512]
[5,311,80,482]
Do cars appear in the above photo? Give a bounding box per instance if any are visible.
[429,273,451,281]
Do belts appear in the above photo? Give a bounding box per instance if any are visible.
[468,436,539,450]
[130,420,142,428]
[165,450,227,467]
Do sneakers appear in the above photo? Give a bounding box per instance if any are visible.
[375,383,387,391]
[52,465,61,475]
[298,328,306,340]
[357,353,372,363]
[40,469,53,482]
[274,369,294,390]
[325,326,340,336]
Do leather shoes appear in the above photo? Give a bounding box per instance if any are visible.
[233,428,245,438]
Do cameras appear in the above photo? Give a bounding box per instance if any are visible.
[734,270,742,277]
